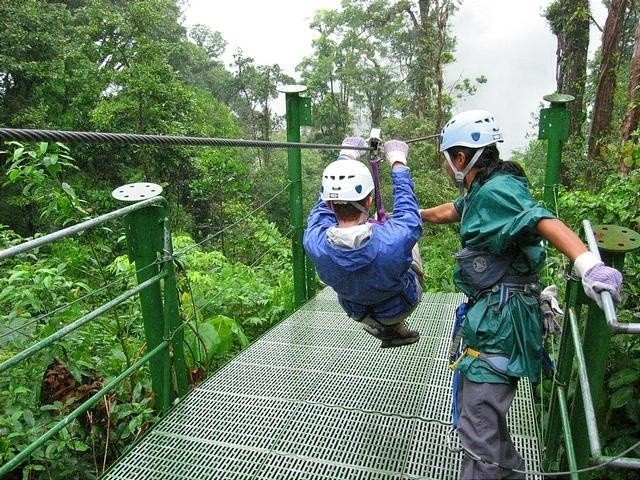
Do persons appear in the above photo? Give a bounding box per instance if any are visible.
[302,136,426,349]
[416,107,624,480]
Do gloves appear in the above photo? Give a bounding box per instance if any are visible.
[340,136,369,160]
[573,251,624,310]
[383,140,409,169]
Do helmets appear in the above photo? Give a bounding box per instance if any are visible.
[439,110,505,152]
[320,160,376,202]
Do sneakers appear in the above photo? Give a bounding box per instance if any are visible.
[379,322,421,349]
